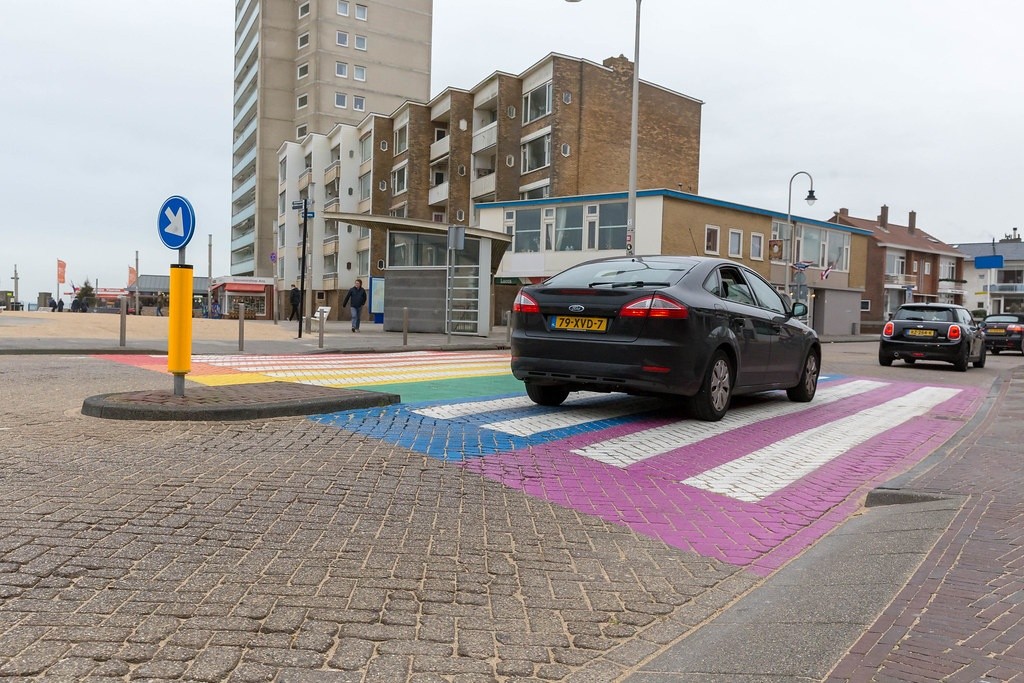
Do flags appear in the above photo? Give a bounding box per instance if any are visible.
[57,260,66,283]
[129,267,136,287]
[819,264,833,280]
[792,260,814,272]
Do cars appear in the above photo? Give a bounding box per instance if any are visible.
[509,253,824,423]
[877,301,988,372]
[983,313,1024,355]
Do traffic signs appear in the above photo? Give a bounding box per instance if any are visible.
[291,201,303,205]
[301,211,315,218]
[291,205,302,210]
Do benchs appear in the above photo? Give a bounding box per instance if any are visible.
[301,306,331,333]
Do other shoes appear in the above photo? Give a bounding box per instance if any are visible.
[355,329,360,333]
[352,327,355,332]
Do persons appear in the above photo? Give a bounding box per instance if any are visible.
[342,280,367,332]
[49,298,64,312]
[287,284,303,322]
[156,291,163,316]
[126,301,129,315]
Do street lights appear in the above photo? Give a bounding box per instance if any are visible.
[563,1,644,256]
[785,171,818,309]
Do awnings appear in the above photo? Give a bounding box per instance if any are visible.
[210,283,224,290]
[224,283,265,293]
